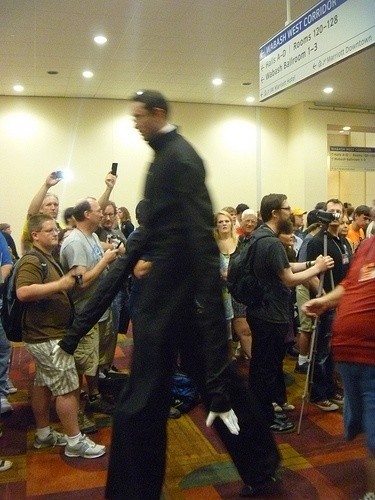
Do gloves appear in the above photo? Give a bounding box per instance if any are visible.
[206,409,240,436]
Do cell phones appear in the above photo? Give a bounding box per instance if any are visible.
[116,240,124,249]
[56,171,63,178]
[111,163,118,175]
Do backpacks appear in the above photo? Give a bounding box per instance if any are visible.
[226,233,278,306]
[0,252,48,343]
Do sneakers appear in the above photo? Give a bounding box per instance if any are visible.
[333,394,345,404]
[312,398,340,411]
[0,399,14,414]
[33,427,67,449]
[64,433,106,459]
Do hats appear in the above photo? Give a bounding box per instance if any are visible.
[291,207,307,215]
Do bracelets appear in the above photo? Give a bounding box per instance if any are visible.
[305,260,311,269]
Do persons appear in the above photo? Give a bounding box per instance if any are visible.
[301,224,375,500]
[101,90,286,500]
[212,193,375,430]
[0,173,183,471]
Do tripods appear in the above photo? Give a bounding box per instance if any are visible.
[297,219,335,435]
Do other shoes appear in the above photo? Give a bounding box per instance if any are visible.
[272,402,282,412]
[7,384,18,393]
[169,407,181,418]
[0,460,13,471]
[78,410,96,433]
[283,403,295,410]
[294,361,309,373]
[86,393,115,414]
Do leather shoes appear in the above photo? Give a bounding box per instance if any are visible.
[271,419,295,432]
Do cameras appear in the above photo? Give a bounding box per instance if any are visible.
[316,210,341,220]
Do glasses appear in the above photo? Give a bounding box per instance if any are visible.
[104,212,114,217]
[361,215,371,222]
[277,206,290,211]
[41,228,60,234]
